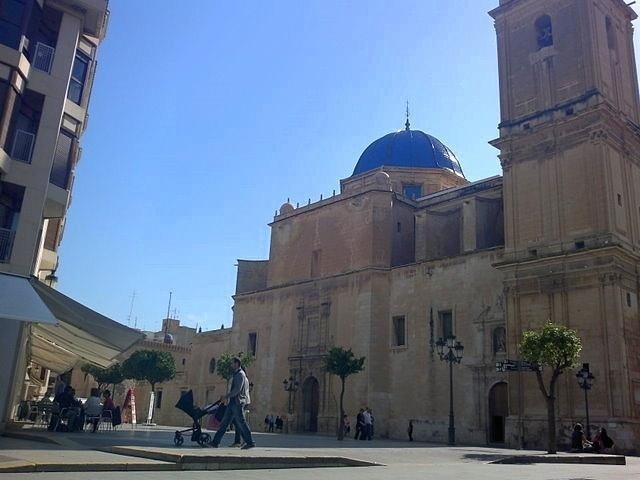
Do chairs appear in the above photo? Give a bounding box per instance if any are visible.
[13,398,116,436]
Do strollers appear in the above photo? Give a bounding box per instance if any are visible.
[174,389,221,447]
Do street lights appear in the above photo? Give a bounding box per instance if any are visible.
[283,376,298,414]
[576,366,595,441]
[435,332,464,445]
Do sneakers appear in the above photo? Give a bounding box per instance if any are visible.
[202,441,256,450]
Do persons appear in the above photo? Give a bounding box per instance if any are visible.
[226,372,239,449]
[263,413,285,433]
[207,358,256,450]
[571,423,601,454]
[342,413,351,437]
[47,382,114,433]
[407,420,414,441]
[590,426,616,454]
[356,408,373,441]
